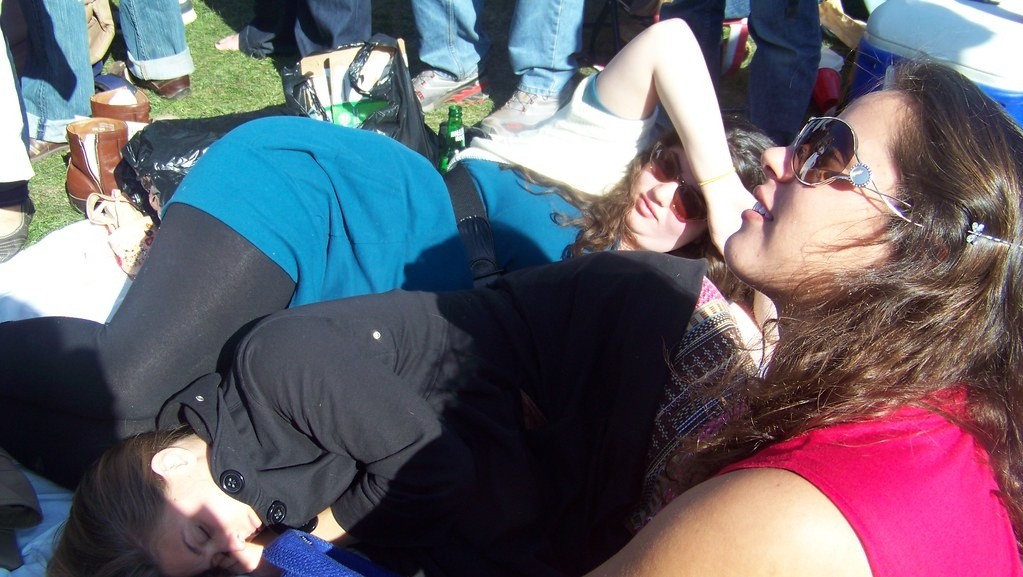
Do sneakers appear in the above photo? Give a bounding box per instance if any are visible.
[408,58,492,111]
[480,88,572,136]
[109,60,190,99]
[24,134,67,161]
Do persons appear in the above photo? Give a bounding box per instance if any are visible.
[0,0,822,266]
[44,252,765,577]
[587,56,1023,577]
[0,17,779,493]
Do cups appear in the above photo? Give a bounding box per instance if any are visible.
[812,68,843,115]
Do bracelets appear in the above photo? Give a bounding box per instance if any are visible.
[699,167,737,188]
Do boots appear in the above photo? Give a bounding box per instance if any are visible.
[65,116,145,214]
[90,85,151,123]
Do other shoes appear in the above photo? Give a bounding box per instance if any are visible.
[0,197,33,262]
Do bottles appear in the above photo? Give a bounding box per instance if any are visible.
[439,105,466,173]
[439,122,448,147]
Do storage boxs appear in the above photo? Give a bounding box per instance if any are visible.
[835,0,1023,133]
[300,37,416,136]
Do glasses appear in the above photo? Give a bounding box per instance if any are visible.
[649,140,706,223]
[791,117,927,230]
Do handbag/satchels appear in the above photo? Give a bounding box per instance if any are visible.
[86,188,160,280]
[278,33,437,163]
